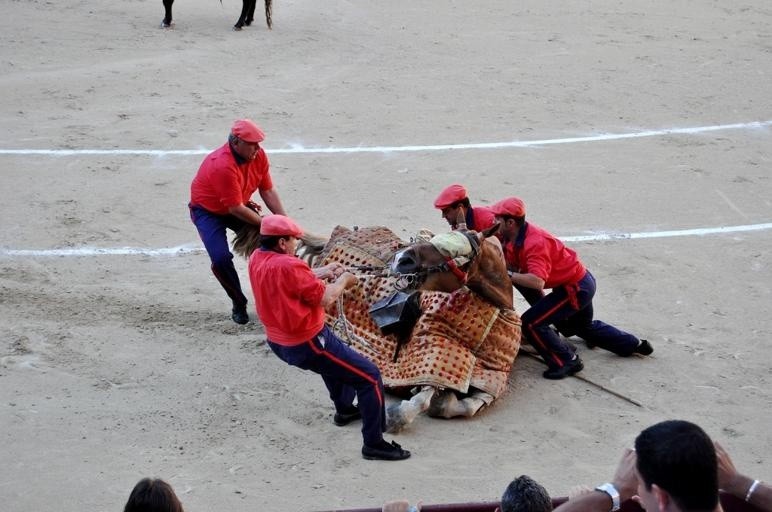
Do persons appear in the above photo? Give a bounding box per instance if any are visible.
[188,118,288,324]
[553,419,772,511]
[489,196,653,378]
[433,183,601,349]
[122,478,184,512]
[382,468,603,511]
[248,214,411,461]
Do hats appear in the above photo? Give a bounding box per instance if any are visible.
[488,197,526,217]
[434,185,468,210]
[232,119,265,143]
[260,214,304,240]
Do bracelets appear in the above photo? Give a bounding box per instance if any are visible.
[595,481,620,511]
[745,477,759,502]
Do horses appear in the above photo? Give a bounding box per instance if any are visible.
[230,207,514,435]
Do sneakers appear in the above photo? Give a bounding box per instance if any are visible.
[362,440,411,460]
[619,340,653,357]
[333,403,362,426]
[543,355,584,379]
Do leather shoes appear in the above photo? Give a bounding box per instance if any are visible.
[232,303,249,324]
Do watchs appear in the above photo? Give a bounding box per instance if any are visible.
[506,270,512,277]
